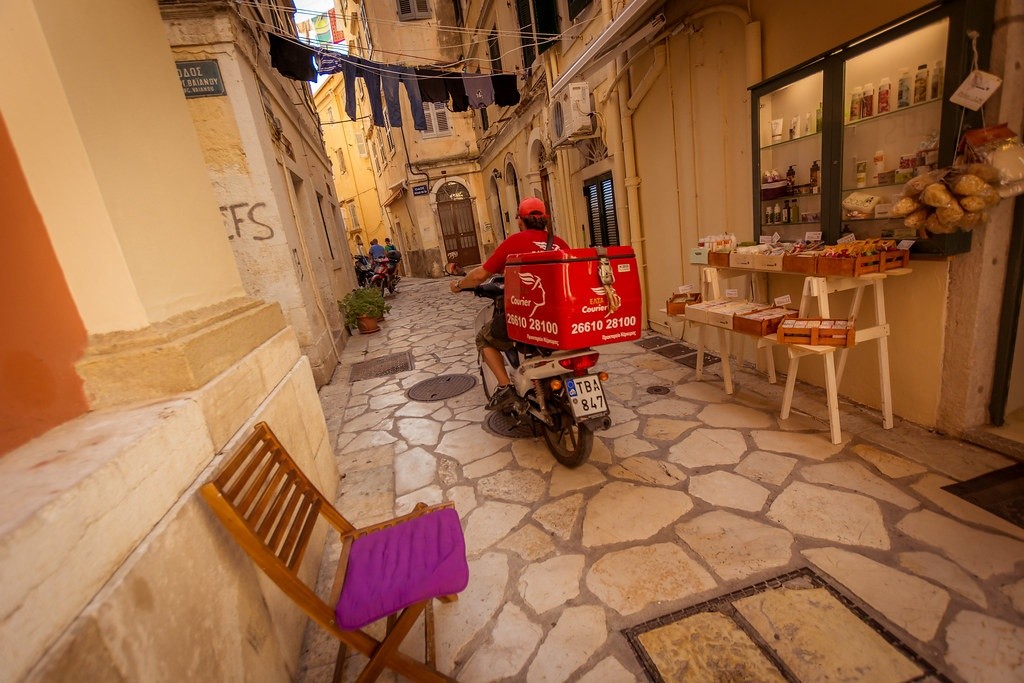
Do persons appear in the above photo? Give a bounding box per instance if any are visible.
[449,198,571,410]
[384,238,395,252]
[369,238,385,259]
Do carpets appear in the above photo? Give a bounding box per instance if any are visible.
[940,458,1024,528]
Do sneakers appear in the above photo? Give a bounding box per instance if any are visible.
[486,383,518,410]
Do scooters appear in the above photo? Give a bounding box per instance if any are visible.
[352,250,401,296]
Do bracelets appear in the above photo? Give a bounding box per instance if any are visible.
[455,281,461,289]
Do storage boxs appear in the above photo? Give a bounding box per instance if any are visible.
[688,239,910,278]
[666,292,799,336]
[760,180,787,202]
[777,316,856,349]
[502,246,642,351]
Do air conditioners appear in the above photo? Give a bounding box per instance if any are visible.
[546,83,593,149]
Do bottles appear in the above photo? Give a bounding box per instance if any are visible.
[782,200,790,223]
[765,206,773,224]
[816,102,823,132]
[790,199,799,224]
[931,61,943,98]
[850,87,862,121]
[862,83,874,119]
[878,77,891,115]
[914,63,928,103]
[898,68,912,108]
[774,203,781,222]
[873,150,884,186]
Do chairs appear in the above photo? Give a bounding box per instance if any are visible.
[199,422,469,683]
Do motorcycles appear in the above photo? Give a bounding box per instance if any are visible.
[444,261,611,468]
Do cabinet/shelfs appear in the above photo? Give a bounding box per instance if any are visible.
[746,0,991,259]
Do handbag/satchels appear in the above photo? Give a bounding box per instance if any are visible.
[490,295,516,342]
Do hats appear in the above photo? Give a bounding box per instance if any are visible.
[518,197,550,219]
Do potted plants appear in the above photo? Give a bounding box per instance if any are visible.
[337,284,392,335]
